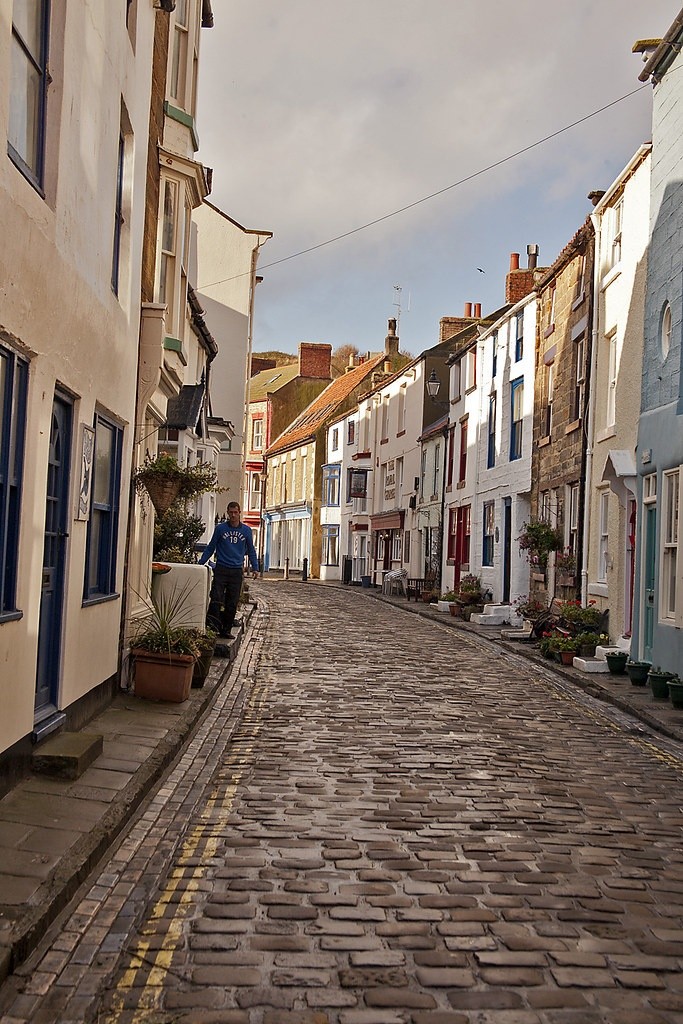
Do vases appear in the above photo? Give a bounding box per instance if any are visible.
[628,664,652,686]
[580,645,596,657]
[552,649,560,662]
[562,650,577,664]
[604,652,630,674]
[669,684,683,710]
[143,478,182,517]
[650,673,678,698]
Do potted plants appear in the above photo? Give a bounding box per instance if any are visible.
[126,583,199,702]
[179,628,217,689]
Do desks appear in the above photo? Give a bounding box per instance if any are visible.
[407,577,437,601]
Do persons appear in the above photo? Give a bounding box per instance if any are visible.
[197,501,259,641]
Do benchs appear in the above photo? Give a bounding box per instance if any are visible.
[522,598,568,638]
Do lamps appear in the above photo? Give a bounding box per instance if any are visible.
[426,367,451,405]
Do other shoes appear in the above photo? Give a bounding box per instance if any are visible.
[219,632,236,639]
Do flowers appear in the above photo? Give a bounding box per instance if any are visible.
[457,574,483,602]
[648,670,673,676]
[513,594,609,644]
[670,677,681,684]
[628,659,649,665]
[134,452,186,483]
[610,648,625,657]
